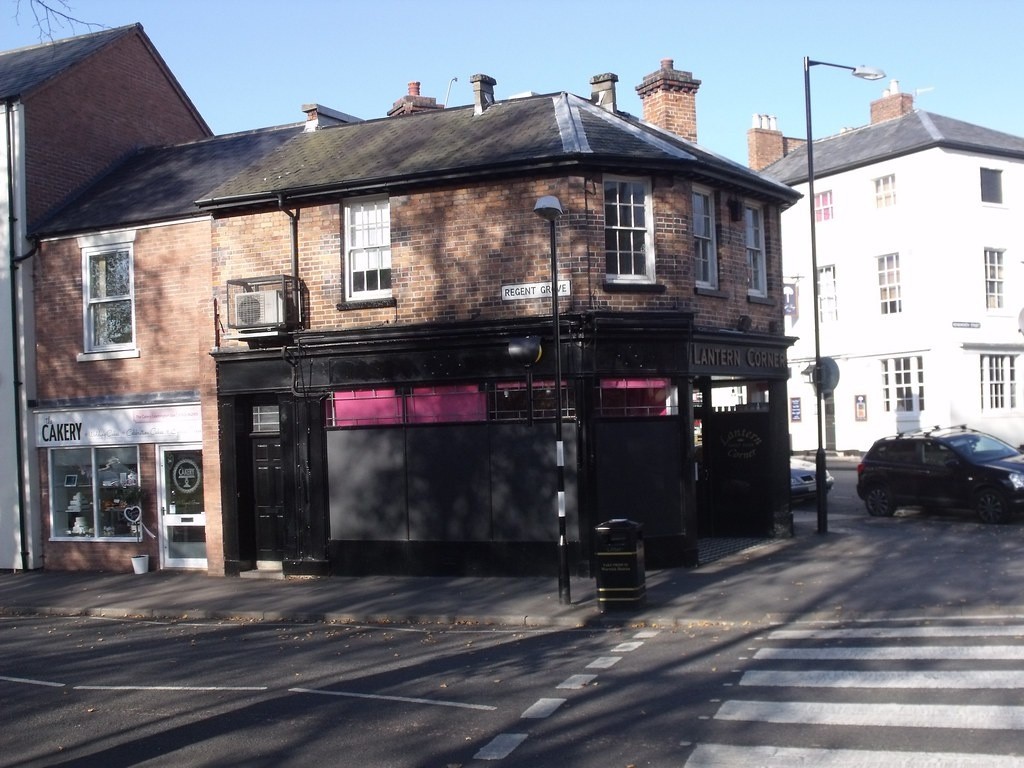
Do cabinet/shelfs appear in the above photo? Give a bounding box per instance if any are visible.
[55,484,123,536]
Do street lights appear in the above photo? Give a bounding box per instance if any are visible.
[805,57,887,535]
[534,196,572,605]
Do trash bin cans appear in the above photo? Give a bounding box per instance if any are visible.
[593,519,645,611]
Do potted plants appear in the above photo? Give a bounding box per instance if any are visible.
[123,486,149,575]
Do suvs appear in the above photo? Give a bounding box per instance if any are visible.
[856,423,1024,525]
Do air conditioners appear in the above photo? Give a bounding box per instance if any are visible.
[232,289,282,326]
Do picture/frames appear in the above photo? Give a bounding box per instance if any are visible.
[64,475,78,488]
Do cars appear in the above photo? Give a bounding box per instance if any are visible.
[694,443,835,507]
